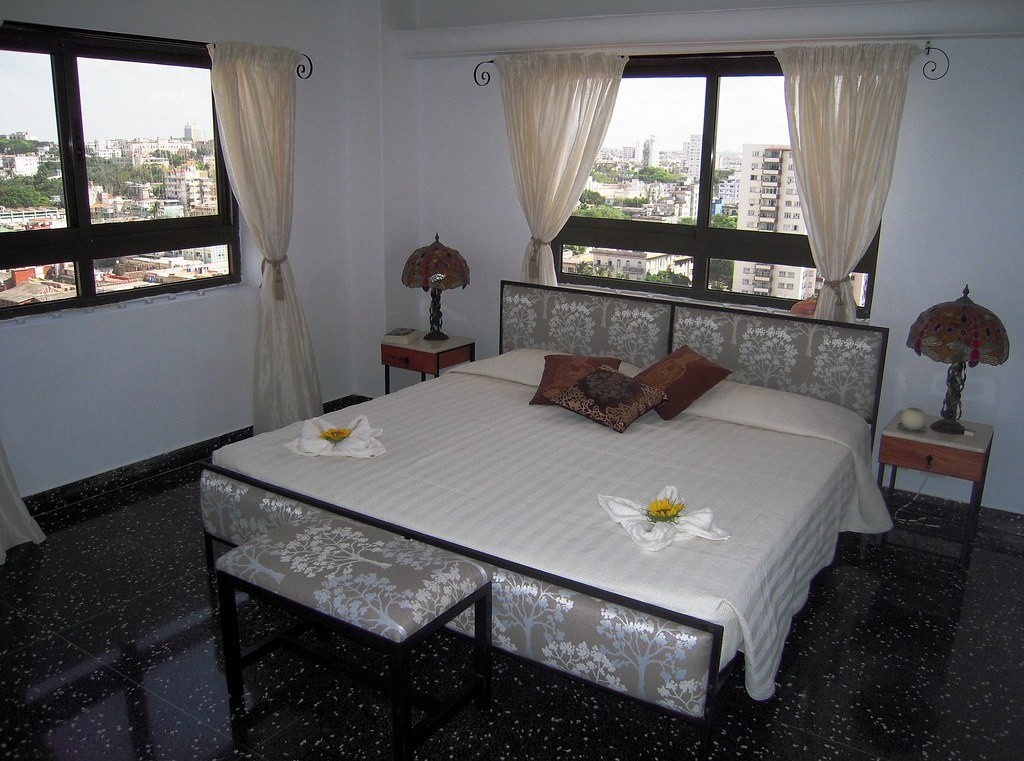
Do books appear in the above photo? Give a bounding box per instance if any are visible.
[384,328,418,343]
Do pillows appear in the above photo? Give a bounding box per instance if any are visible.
[551,367,663,433]
[529,354,625,404]
[635,345,730,421]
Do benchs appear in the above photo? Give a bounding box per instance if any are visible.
[215,515,492,756]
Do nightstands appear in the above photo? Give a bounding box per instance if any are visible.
[866,409,997,568]
[380,328,476,396]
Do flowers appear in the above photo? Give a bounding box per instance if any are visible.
[639,497,684,526]
[318,426,352,447]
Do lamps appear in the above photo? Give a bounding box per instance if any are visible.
[401,233,469,341]
[907,283,1010,434]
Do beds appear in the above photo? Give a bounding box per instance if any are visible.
[199,279,890,718]
